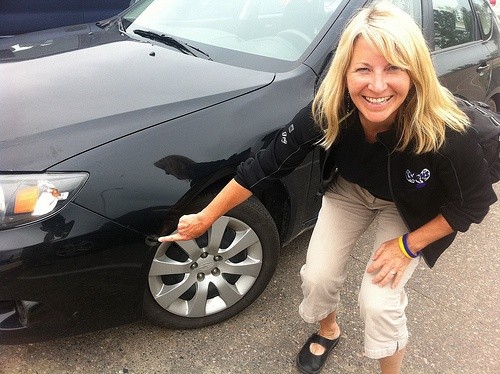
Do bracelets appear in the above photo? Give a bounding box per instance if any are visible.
[397,235,415,260]
[401,233,419,258]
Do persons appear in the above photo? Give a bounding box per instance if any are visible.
[154,3,499,373]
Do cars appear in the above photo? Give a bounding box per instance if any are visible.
[0,0,500,345]
[20,206,184,268]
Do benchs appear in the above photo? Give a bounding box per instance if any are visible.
[433,9,473,51]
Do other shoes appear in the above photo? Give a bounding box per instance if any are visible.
[296,331,341,374]
